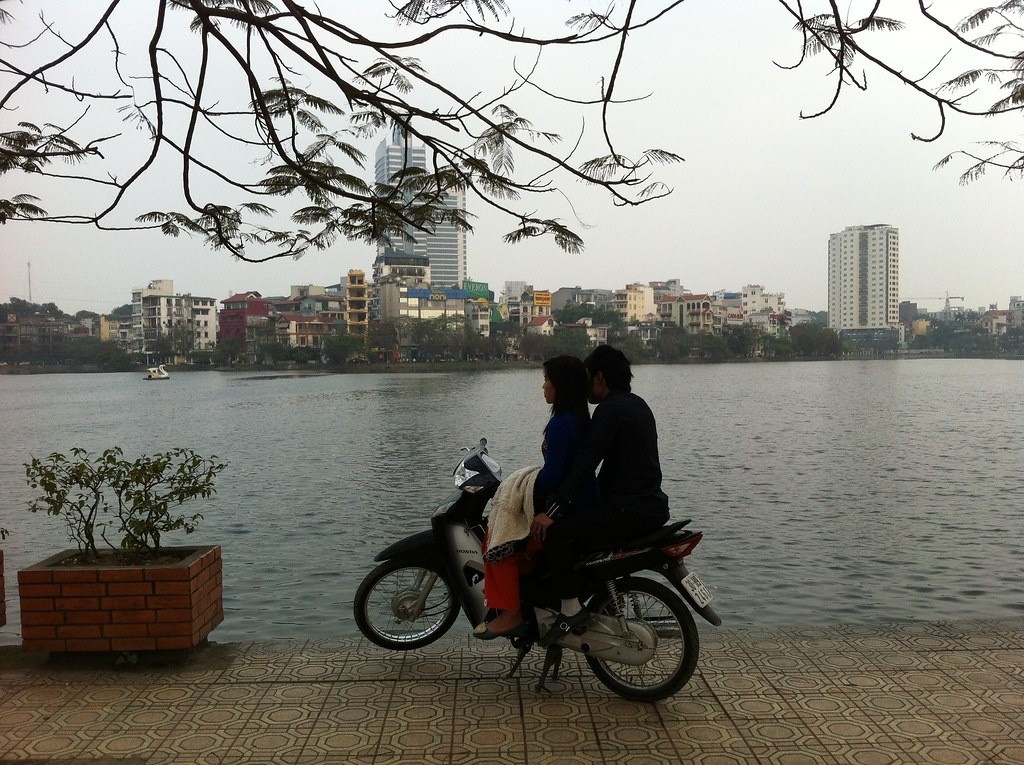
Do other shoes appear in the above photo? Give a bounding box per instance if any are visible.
[539,604,593,643]
[472,608,537,639]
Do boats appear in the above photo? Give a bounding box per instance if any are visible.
[143,364,170,380]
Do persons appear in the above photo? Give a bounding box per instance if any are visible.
[473,353,597,639]
[529,344,670,647]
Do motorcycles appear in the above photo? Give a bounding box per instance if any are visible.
[352,437,723,703]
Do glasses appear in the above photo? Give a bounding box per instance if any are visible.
[585,368,602,384]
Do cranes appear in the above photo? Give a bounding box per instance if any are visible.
[900,291,965,305]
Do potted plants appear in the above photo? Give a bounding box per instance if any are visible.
[16,446,230,658]
